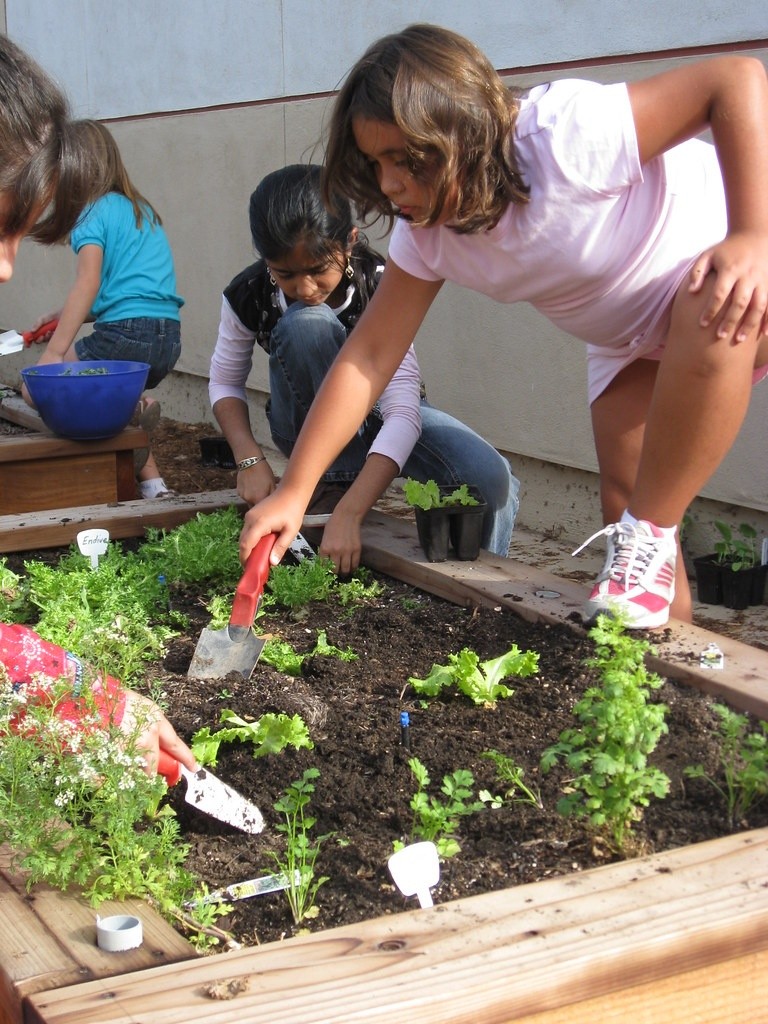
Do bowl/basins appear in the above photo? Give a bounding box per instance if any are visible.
[20,360,151,441]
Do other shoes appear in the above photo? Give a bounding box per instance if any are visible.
[141,491,181,500]
[130,397,162,477]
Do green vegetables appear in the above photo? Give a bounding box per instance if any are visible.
[402,475,481,511]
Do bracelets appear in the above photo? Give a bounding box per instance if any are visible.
[237,456,267,470]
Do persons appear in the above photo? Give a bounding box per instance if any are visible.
[23,120,185,500]
[209,163,524,556]
[0,33,105,283]
[239,20,768,629]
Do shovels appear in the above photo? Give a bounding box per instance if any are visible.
[187,533,279,679]
[289,533,317,564]
[155,752,265,835]
[0,320,58,357]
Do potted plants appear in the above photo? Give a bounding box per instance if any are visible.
[692,521,768,610]
[20,360,151,441]
[399,476,491,563]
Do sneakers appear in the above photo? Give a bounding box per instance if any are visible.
[571,509,677,629]
[303,482,349,528]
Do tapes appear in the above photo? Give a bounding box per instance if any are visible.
[96,914,143,952]
